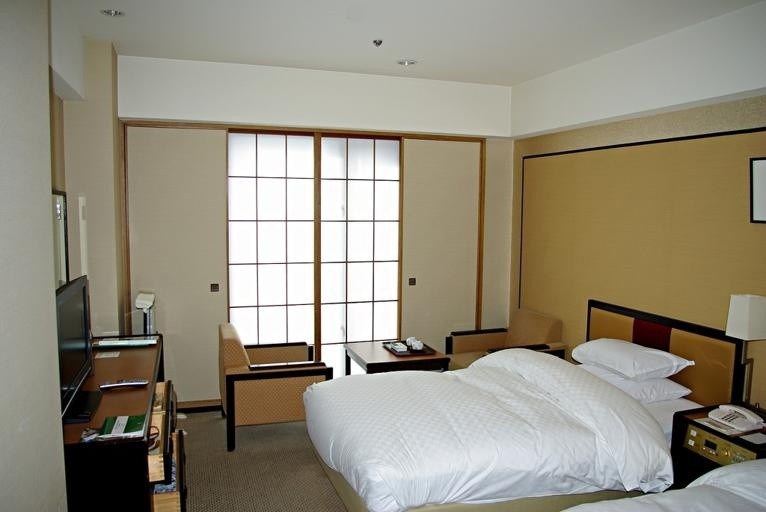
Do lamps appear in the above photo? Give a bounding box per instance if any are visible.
[723,292,766,416]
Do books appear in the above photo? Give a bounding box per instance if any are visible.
[99,414,146,438]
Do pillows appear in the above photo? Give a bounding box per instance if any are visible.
[576,360,693,406]
[571,335,696,385]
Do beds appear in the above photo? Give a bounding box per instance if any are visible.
[300,296,753,512]
[557,455,766,511]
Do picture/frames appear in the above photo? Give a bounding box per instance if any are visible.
[748,156,766,224]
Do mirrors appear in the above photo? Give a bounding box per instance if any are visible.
[50,190,69,292]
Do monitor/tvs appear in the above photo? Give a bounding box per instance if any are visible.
[54,274,103,423]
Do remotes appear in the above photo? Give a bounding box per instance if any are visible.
[99,378,149,390]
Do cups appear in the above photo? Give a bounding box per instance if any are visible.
[406,336,423,350]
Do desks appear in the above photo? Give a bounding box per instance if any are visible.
[340,338,452,379]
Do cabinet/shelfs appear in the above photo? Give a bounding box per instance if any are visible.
[60,332,188,511]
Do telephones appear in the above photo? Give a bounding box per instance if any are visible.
[708,404,766,432]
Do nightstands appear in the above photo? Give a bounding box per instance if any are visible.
[672,400,766,487]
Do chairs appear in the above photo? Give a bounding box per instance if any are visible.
[217,320,334,452]
[443,305,570,375]
